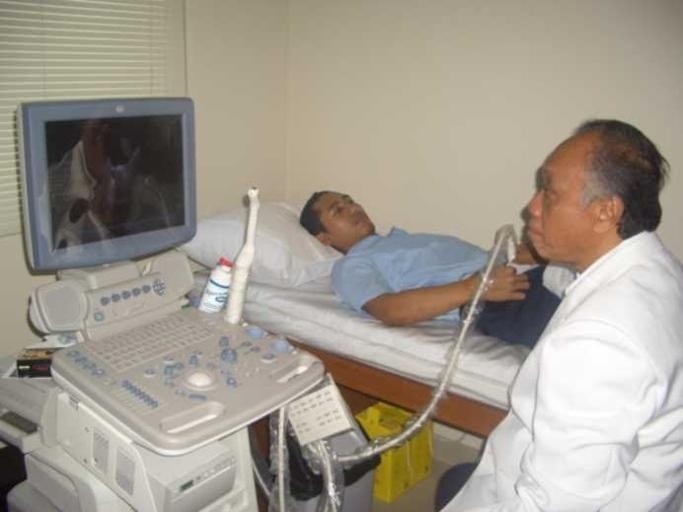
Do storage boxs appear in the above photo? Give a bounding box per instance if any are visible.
[352,403,435,501]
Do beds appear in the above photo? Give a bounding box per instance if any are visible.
[186,199,530,437]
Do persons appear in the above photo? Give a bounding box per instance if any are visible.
[299,190,563,351]
[434,119,682,512]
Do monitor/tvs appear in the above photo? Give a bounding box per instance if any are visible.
[20,95,199,272]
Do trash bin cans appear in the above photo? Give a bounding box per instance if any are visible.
[270,411,382,512]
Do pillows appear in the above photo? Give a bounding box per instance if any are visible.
[173,198,379,290]
